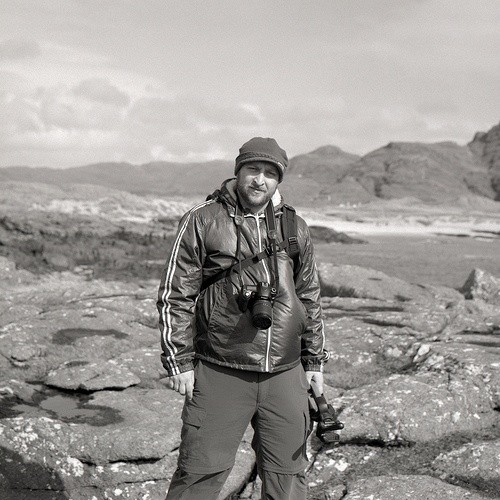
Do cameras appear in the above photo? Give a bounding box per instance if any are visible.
[238,281,277,330]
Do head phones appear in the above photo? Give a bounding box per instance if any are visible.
[310,380,343,443]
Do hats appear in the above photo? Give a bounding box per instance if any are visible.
[234,137,288,184]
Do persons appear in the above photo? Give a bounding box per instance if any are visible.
[156,137,326,500]
